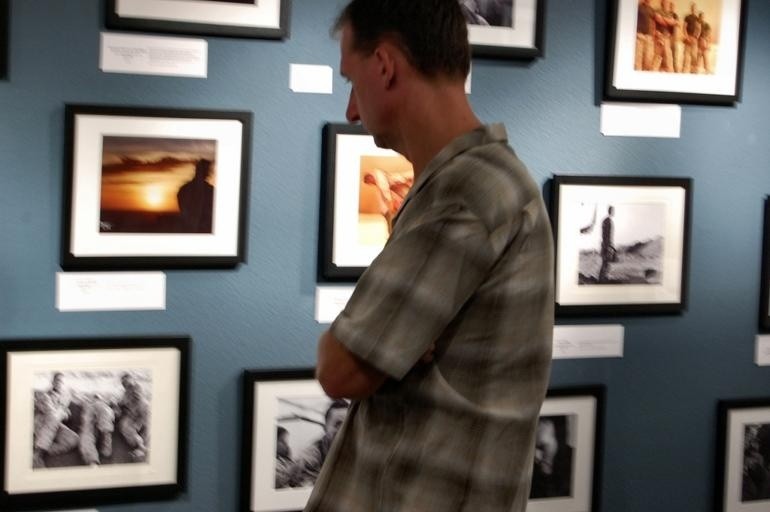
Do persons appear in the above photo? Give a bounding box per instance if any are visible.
[595,204,617,286]
[300,1,558,512]
[72,379,125,467]
[114,372,149,461]
[531,416,575,499]
[636,2,718,75]
[33,370,80,471]
[275,424,296,486]
[174,155,213,230]
[291,398,352,487]
